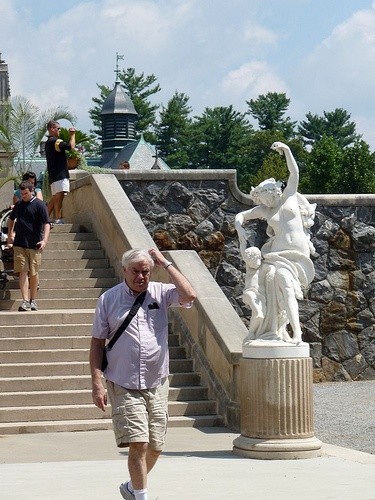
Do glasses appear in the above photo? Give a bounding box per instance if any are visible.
[52,127,60,130]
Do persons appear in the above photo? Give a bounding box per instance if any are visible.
[89,247,196,500]
[118,160,131,170]
[7,180,51,313]
[45,119,77,224]
[234,141,316,344]
[8,169,45,209]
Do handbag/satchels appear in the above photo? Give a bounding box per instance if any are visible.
[100,345,108,372]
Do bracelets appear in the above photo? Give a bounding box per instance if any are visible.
[163,261,173,269]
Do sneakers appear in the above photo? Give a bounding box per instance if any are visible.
[120,482,136,500]
[30,300,38,311]
[18,301,30,310]
[49,218,64,224]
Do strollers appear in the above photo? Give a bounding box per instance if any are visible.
[0,208,17,289]
